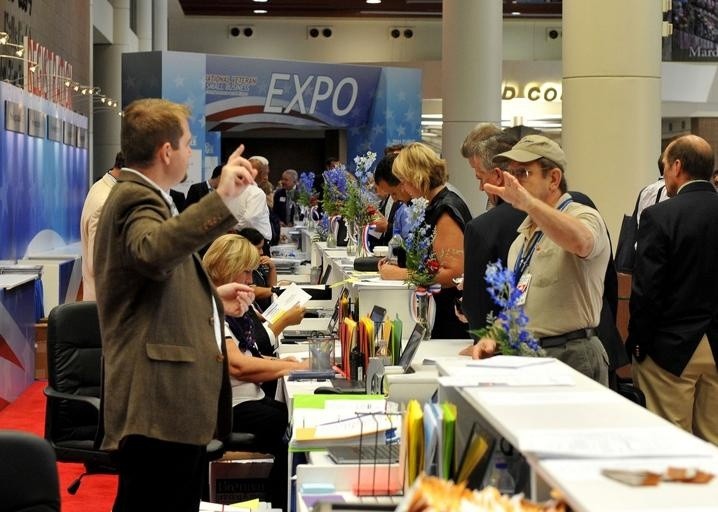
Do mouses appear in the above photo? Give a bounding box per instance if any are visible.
[314,386,341,395]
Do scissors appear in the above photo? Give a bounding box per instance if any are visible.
[310,331,325,345]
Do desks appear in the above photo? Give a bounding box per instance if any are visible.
[268,221,717,512]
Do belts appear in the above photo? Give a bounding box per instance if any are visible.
[538,326,599,350]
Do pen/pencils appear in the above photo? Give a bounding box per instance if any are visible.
[310,338,333,372]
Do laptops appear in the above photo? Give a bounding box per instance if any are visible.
[328,389,438,465]
[370,307,386,340]
[320,262,332,287]
[330,323,426,393]
[283,288,349,338]
[449,422,496,488]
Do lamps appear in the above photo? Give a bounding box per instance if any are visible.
[0,31,126,120]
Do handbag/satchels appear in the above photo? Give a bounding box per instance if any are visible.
[613,214,641,274]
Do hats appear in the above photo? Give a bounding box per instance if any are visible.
[492,135,566,172]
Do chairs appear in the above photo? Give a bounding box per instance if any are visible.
[232,433,268,452]
[44,299,119,493]
[2,426,65,511]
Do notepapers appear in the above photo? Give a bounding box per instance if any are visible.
[300,482,335,494]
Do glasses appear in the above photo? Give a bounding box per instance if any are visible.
[504,165,553,180]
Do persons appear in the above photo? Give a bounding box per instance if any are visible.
[634,146,671,222]
[626,135,718,449]
[187,155,300,338]
[470,135,613,389]
[80,149,131,302]
[326,157,340,170]
[712,170,718,188]
[197,234,309,511]
[90,95,259,511]
[459,134,631,390]
[373,122,545,339]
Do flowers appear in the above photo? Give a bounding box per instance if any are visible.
[296,151,380,224]
[405,197,458,291]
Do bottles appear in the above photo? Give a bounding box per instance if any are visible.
[488,462,516,495]
[388,229,403,265]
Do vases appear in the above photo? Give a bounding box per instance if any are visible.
[303,209,373,259]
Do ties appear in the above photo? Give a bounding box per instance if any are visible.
[286,191,293,225]
